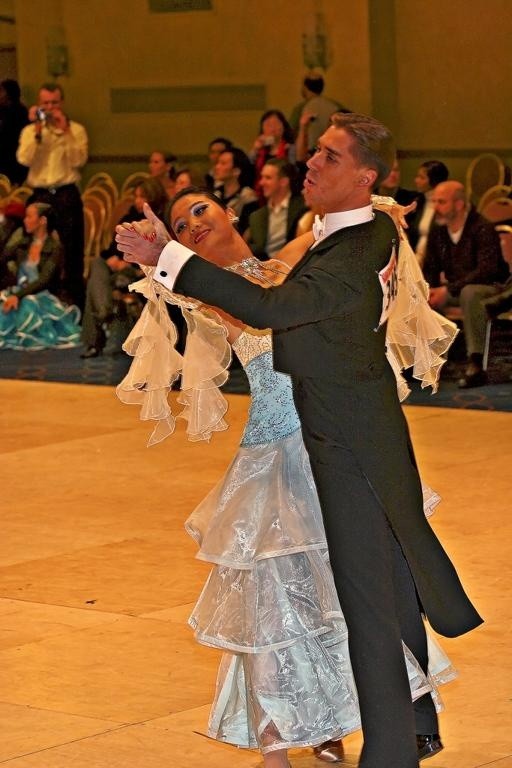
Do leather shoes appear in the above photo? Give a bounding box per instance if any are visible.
[458,370,486,388]
[413,732,444,761]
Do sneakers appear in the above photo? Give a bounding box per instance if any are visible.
[314,740,347,765]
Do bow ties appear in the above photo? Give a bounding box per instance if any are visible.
[311,214,324,241]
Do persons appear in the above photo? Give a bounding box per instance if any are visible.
[14,81,89,332]
[422,181,512,388]
[1,109,451,357]
[115,184,462,768]
[287,73,350,182]
[114,113,485,768]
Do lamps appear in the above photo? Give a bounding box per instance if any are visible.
[46,41,67,76]
[301,14,323,68]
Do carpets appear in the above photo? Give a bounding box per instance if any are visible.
[1,336,512,412]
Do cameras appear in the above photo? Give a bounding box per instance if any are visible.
[35,108,52,122]
